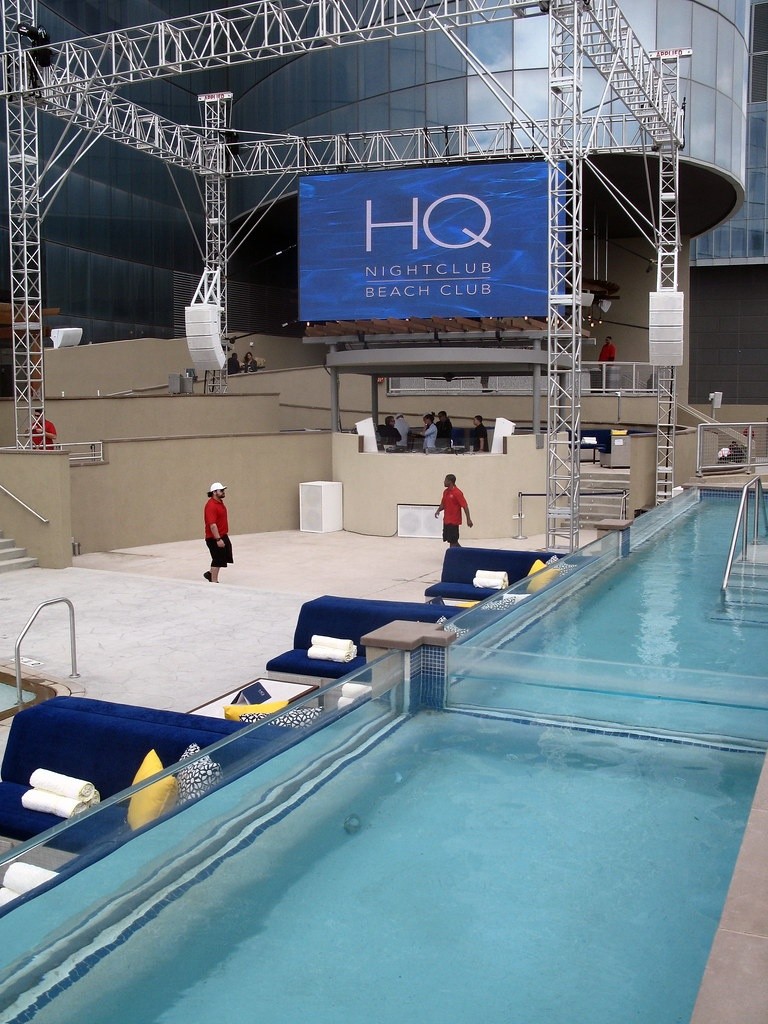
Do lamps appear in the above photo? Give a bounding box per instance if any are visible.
[591,303,594,327]
[598,297,603,324]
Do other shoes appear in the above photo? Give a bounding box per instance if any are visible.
[203,571,219,583]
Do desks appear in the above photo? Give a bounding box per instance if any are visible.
[186,677,325,720]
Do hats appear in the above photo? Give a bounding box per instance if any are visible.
[210,482,227,493]
[395,414,403,418]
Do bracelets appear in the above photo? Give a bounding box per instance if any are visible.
[480,448,483,450]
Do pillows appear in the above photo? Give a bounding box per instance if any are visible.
[456,602,479,608]
[126,749,177,832]
[545,555,578,579]
[473,597,515,610]
[610,429,627,435]
[224,701,289,721]
[527,559,561,593]
[174,744,223,809]
[240,705,324,730]
[436,616,469,639]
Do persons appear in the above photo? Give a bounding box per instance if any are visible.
[598,336,615,393]
[435,411,453,448]
[435,474,474,547]
[471,415,490,453]
[379,413,410,451]
[647,374,657,393]
[24,409,57,450]
[420,412,438,453]
[243,352,258,373]
[203,483,234,583]
[719,426,754,464]
[228,353,241,376]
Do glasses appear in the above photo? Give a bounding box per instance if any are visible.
[34,414,41,417]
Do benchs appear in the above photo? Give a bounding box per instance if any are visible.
[0,519,604,874]
[564,429,656,469]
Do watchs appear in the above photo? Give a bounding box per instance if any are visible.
[215,537,221,541]
